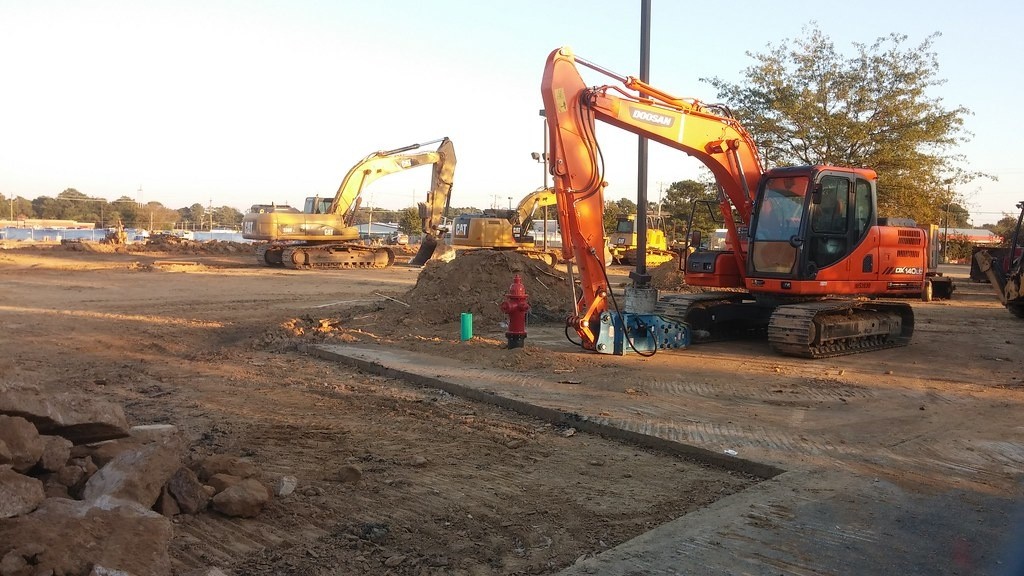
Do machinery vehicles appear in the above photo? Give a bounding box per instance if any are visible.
[974,200,1024,323]
[99,217,128,247]
[607,212,679,267]
[915,223,957,302]
[671,241,696,258]
[540,45,928,357]
[450,187,613,271]
[242,137,458,270]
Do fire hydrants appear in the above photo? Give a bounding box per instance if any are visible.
[500,274,530,349]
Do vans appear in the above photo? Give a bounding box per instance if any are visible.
[712,228,730,251]
